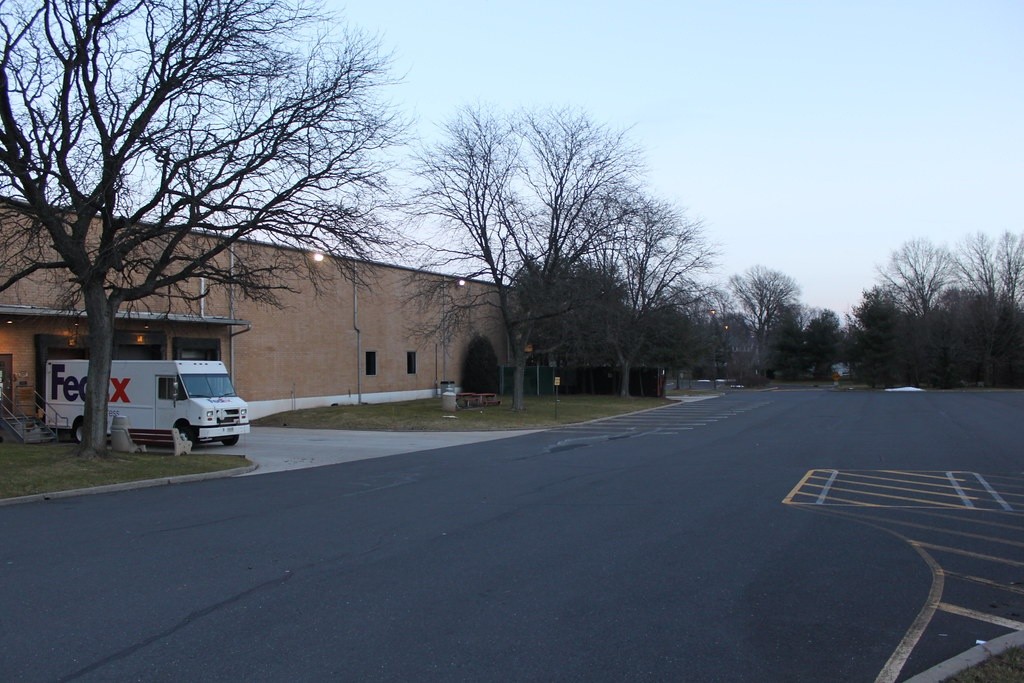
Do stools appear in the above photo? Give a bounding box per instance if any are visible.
[465,398,482,407]
[485,400,501,406]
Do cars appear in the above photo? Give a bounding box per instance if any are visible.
[831,362,859,382]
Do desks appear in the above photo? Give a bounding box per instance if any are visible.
[456,393,496,409]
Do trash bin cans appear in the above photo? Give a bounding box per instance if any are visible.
[441,381,455,396]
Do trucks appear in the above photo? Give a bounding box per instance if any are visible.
[43,357,250,447]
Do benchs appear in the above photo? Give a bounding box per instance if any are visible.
[122,427,192,456]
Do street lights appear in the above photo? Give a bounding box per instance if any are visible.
[724,324,730,385]
[709,308,717,388]
[443,279,466,379]
